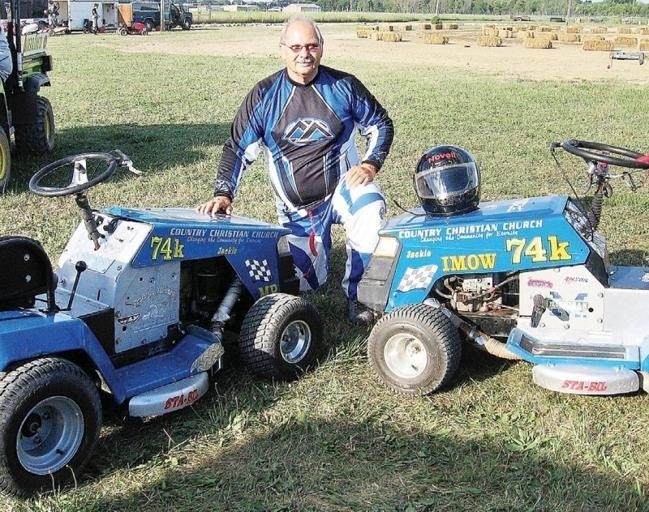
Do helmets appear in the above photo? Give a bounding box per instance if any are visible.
[411,144,482,216]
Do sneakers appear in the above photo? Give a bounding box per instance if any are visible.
[347,299,374,328]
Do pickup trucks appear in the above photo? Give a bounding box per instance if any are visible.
[130,1,193,32]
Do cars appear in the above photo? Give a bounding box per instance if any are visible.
[356,137,649,399]
[0,1,56,193]
[118,20,148,36]
[0,150,325,502]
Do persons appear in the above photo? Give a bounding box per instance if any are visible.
[92,4,100,35]
[196,17,393,326]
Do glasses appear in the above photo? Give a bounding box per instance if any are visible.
[281,42,322,54]
